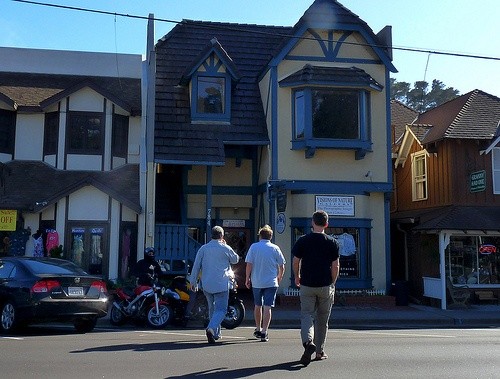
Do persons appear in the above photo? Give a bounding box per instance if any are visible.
[134,246,162,277]
[190,226,239,345]
[186,272,201,317]
[49,248,56,256]
[292,210,340,366]
[245,224,287,342]
[0,237,11,256]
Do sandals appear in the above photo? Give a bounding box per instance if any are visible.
[300,341,316,366]
[315,352,329,359]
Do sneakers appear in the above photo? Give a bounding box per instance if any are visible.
[252,329,261,339]
[261,331,269,341]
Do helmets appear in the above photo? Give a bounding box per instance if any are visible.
[145,247,154,253]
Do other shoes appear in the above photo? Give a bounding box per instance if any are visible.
[205,328,216,344]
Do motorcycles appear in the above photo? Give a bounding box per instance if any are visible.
[165,275,246,331]
[110,265,175,327]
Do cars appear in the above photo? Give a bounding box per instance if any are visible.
[0,256,110,333]
